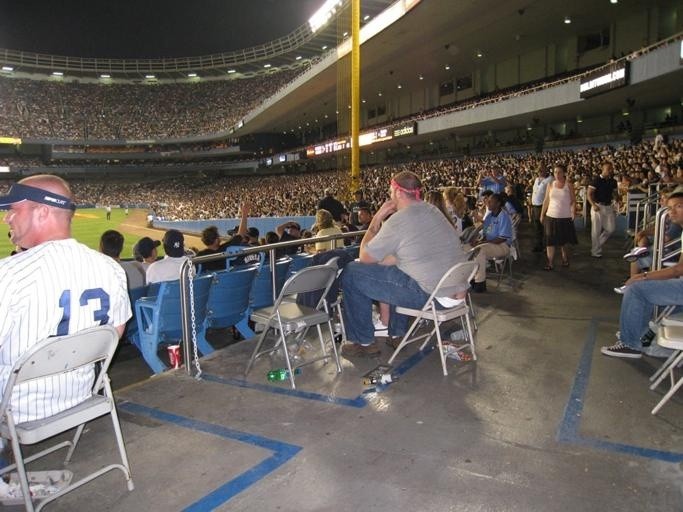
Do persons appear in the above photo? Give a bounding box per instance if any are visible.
[600,192,683,359]
[342,171,471,357]
[1,0,682,290]
[0,174,132,475]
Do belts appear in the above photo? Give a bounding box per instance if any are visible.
[597,201,612,206]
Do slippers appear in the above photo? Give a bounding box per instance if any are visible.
[562,256,569,267]
[544,264,552,270]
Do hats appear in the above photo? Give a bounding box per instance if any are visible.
[0,182,77,211]
[163,229,185,258]
[137,236,161,256]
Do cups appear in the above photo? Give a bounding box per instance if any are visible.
[167,345,182,369]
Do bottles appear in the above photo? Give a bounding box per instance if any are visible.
[364,373,400,385]
[267,368,301,382]
[450,330,469,341]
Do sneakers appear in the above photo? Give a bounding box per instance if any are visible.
[384,334,408,352]
[613,285,627,295]
[601,342,642,359]
[342,343,381,360]
[624,247,649,263]
[373,318,396,339]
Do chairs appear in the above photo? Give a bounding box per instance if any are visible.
[243,264,342,392]
[195,267,256,356]
[650,312,683,382]
[0,323,135,511]
[290,257,314,273]
[126,284,148,319]
[344,245,359,260]
[146,279,179,303]
[294,257,347,362]
[235,259,292,339]
[648,326,683,415]
[491,228,515,288]
[125,274,213,373]
[387,262,480,376]
